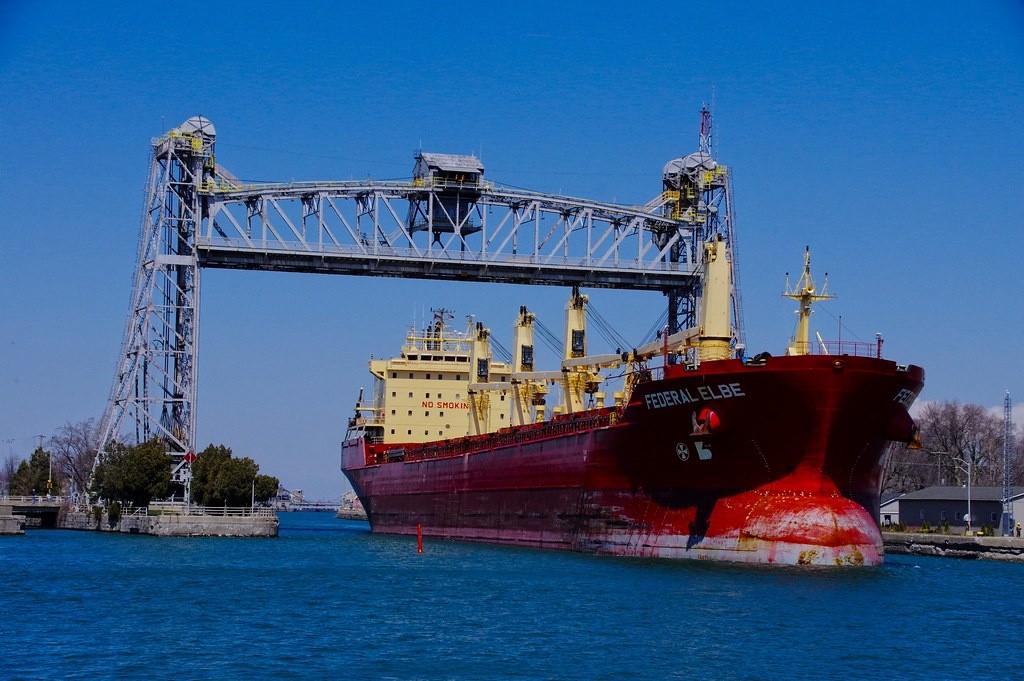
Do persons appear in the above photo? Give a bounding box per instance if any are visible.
[31,489,36,503]
[1016,523,1022,537]
[1009,524,1014,536]
[905,536,915,550]
[943,541,951,549]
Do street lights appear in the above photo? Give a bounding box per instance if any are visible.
[952,457,971,530]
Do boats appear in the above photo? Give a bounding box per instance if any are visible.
[335,491,369,519]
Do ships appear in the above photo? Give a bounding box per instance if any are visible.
[337,224,930,573]
